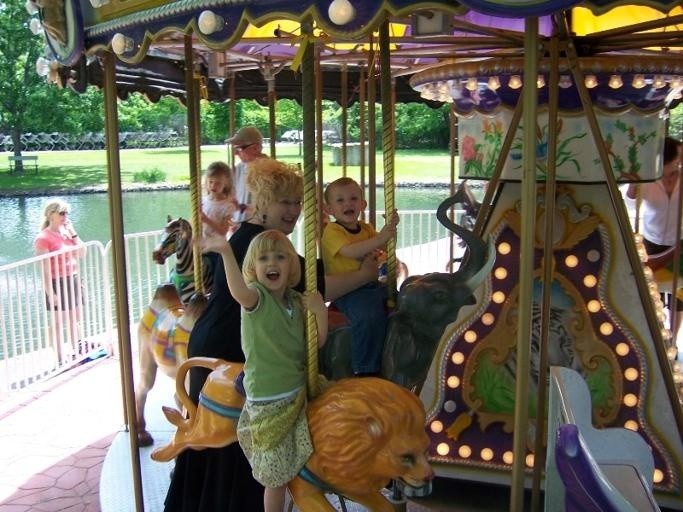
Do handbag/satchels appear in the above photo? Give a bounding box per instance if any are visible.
[70,235,79,238]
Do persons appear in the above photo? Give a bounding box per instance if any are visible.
[201,160,238,270]
[160,156,384,512]
[623,137,681,358]
[322,173,385,377]
[222,125,268,235]
[40,202,90,363]
[185,220,328,511]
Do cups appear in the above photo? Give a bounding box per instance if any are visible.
[236,143,254,152]
[59,211,67,216]
[278,199,304,208]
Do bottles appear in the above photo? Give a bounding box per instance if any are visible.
[223,127,261,144]
